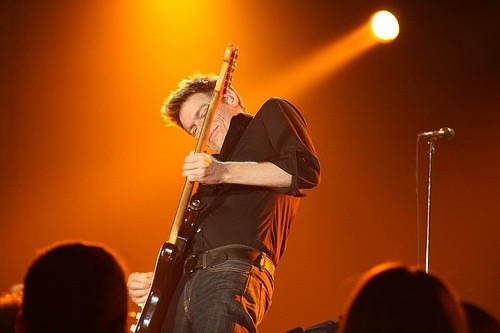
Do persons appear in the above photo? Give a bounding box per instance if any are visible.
[126,73,321,333]
[338,263,468,333]
[0,241,129,333]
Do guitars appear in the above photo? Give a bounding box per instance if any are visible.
[129,44,240,333]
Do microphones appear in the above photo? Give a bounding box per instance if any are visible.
[420,128,455,138]
